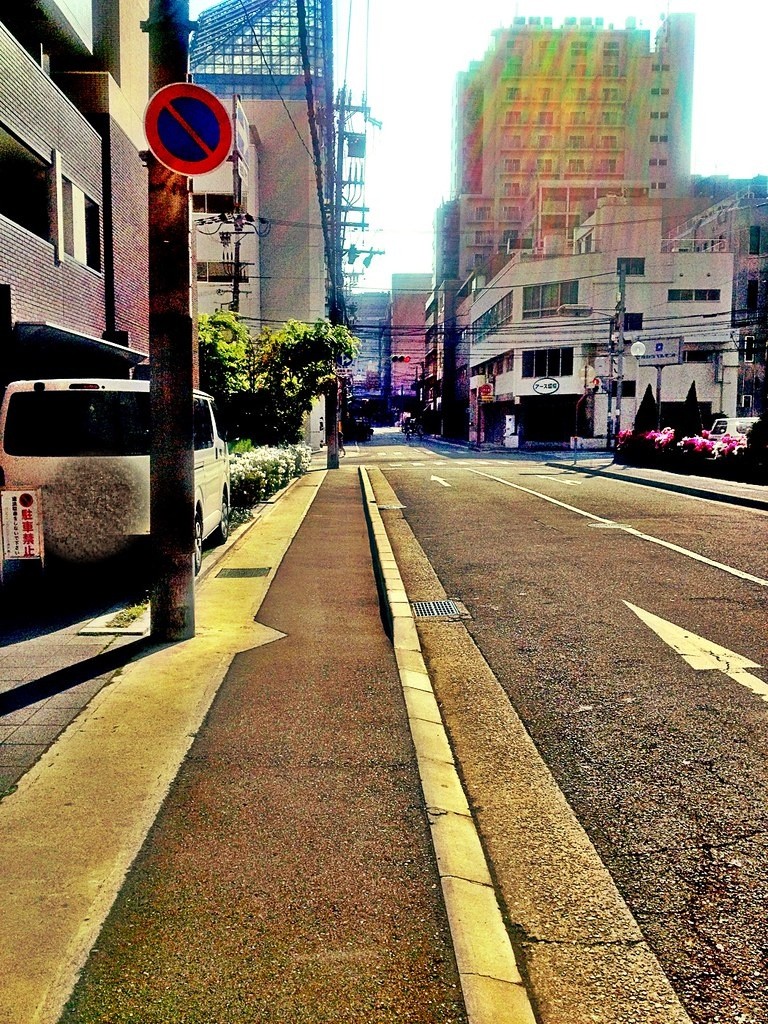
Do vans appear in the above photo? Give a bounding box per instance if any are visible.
[0,379,230,577]
[708,417,760,442]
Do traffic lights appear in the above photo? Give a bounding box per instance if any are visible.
[392,356,411,363]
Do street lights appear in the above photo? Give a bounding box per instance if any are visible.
[558,304,615,449]
